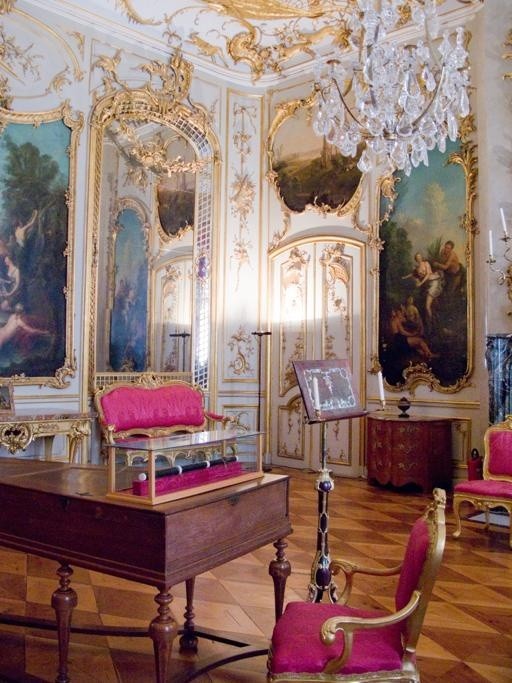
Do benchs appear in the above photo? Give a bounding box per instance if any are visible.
[88,375,241,461]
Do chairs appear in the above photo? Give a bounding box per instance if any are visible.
[263,485,448,683]
[450,415,512,540]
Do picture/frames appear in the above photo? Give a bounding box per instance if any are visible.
[0,84,86,392]
[264,86,371,217]
[103,197,155,374]
[152,130,194,243]
[368,73,479,399]
[0,381,17,417]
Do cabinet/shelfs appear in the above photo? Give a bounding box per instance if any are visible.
[105,426,268,506]
[364,409,454,494]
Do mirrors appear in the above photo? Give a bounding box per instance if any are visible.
[87,89,217,401]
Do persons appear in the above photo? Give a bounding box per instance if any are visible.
[390,309,431,356]
[432,240,465,292]
[401,296,423,335]
[0,208,49,351]
[402,252,443,318]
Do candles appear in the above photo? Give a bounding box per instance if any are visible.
[486,224,492,259]
[311,374,319,411]
[498,208,507,237]
[376,370,385,402]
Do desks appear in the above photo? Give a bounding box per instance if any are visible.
[1,417,93,464]
[0,453,296,682]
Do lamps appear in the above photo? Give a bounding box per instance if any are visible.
[312,0,471,185]
